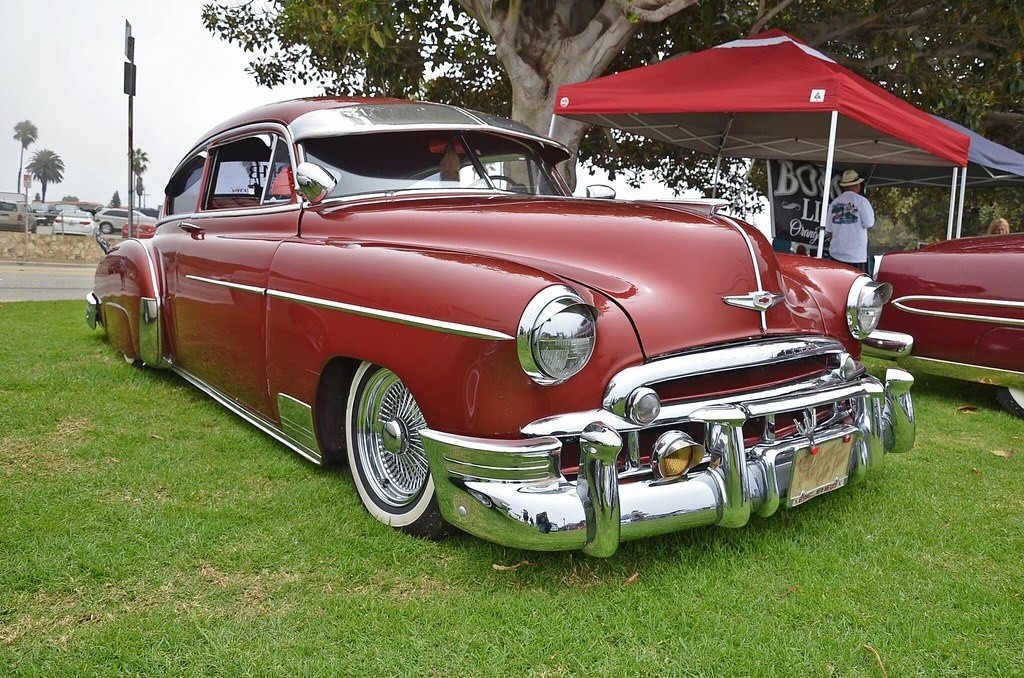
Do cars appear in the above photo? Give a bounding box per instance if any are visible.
[0,202,158,241]
[87,95,914,559]
[860,233,1024,420]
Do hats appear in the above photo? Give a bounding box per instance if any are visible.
[839,170,865,187]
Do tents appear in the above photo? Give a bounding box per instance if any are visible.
[832,112,1024,239]
[538,28,972,259]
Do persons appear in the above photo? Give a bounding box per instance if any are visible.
[826,169,875,273]
[986,218,1010,236]
